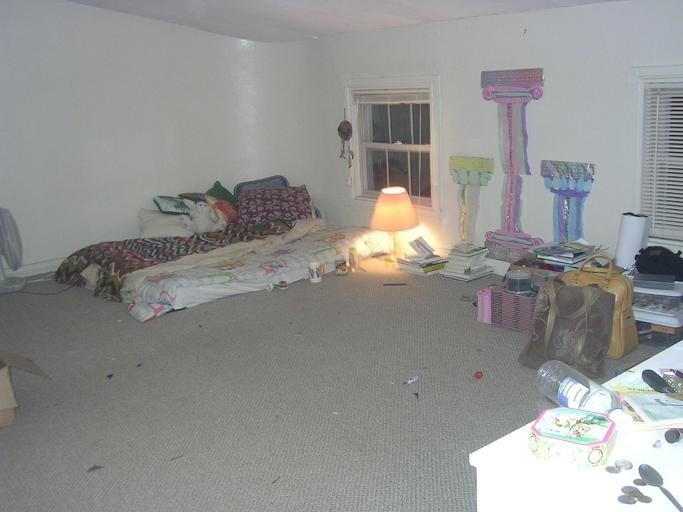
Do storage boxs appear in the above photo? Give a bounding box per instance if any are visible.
[0,349,52,428]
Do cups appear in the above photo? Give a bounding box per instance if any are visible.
[307,261,322,284]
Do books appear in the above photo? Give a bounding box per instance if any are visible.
[558,250,584,258]
[535,238,610,266]
[397,234,497,284]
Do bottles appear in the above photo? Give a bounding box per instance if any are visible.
[532,358,632,431]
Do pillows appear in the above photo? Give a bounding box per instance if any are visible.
[138,175,322,238]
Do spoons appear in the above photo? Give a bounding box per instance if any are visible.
[638,460,683,512]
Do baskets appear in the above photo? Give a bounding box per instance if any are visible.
[475,286,536,334]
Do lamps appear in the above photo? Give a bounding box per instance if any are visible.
[370,187,420,262]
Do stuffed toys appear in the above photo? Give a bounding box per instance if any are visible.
[180,198,227,235]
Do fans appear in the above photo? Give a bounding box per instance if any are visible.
[0,208,26,295]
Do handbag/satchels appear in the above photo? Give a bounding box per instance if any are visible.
[565,256,641,358]
[520,279,617,375]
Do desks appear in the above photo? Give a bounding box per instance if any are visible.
[469,340,683,512]
